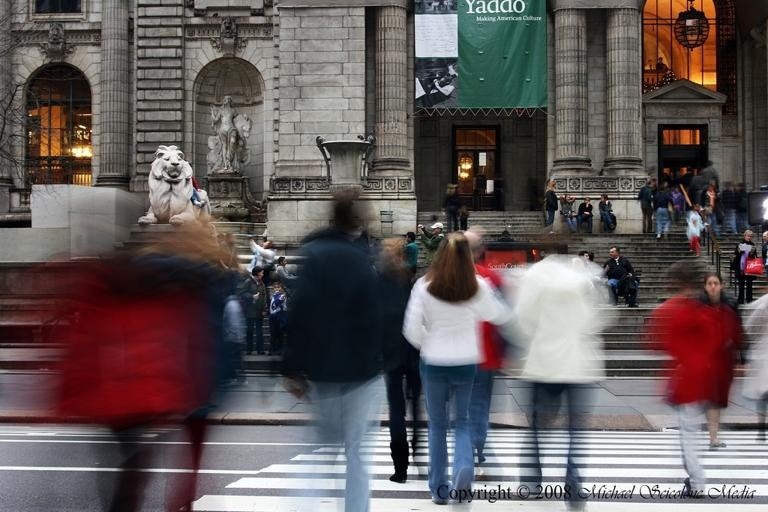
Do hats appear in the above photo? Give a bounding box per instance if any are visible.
[430,222,445,230]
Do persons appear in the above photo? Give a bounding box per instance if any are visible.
[206,95,239,168]
[45,155,768,512]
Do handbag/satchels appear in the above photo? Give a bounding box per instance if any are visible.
[744,255,765,275]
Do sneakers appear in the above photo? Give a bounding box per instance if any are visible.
[389,472,408,483]
